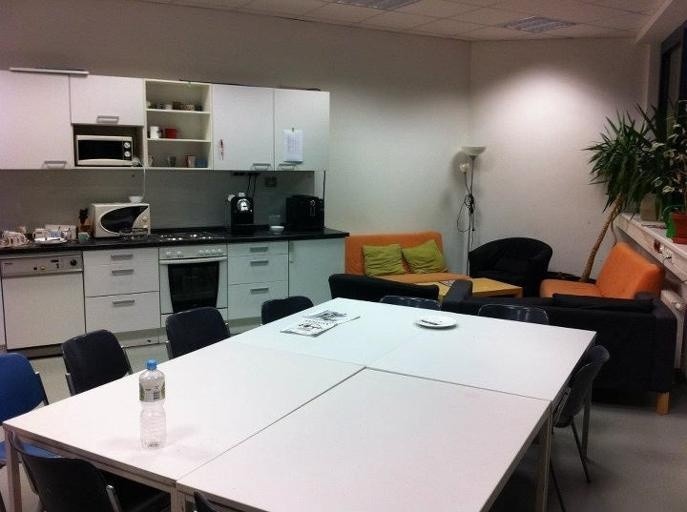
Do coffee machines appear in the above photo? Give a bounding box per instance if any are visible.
[231,192,253,232]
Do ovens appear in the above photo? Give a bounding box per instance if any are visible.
[160,257,229,325]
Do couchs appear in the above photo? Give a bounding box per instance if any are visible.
[538,242,663,304]
[441,278,678,416]
[328,273,473,310]
[343,231,471,284]
[465,237,553,297]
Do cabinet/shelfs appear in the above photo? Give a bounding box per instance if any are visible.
[81,247,160,335]
[227,239,288,321]
[145,79,212,170]
[288,238,345,305]
[70,74,146,127]
[0,71,74,171]
[211,84,329,171]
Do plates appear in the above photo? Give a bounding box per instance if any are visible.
[415,315,457,329]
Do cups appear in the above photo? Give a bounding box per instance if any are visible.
[185,155,197,167]
[185,104,194,110]
[1,223,76,246]
[77,232,89,244]
[173,102,184,110]
[164,104,172,109]
[166,128,177,138]
[166,153,176,166]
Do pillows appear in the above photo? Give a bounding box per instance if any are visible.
[361,243,405,277]
[401,239,448,274]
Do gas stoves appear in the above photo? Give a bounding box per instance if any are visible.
[155,230,225,242]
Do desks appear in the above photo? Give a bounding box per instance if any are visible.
[0,297,596,511]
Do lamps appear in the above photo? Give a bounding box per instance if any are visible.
[458,146,485,275]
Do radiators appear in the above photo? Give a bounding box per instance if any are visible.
[659,289,685,367]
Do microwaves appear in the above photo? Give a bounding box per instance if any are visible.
[76,135,134,166]
[91,202,152,239]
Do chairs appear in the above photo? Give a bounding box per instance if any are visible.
[6,430,170,512]
[546,345,609,511]
[60,330,135,396]
[0,351,52,512]
[261,295,313,327]
[380,295,442,312]
[476,304,549,324]
[163,307,231,360]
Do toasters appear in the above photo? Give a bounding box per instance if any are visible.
[286,195,325,229]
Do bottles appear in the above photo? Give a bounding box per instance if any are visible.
[139,360,168,448]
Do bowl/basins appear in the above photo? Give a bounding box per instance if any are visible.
[128,196,144,203]
[270,225,284,234]
